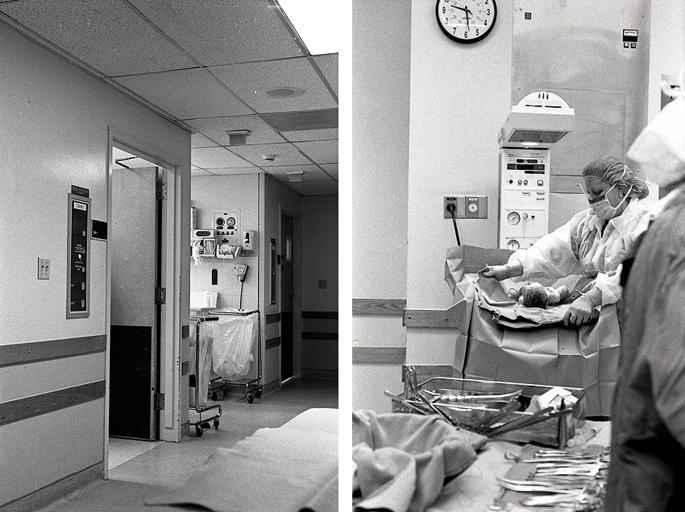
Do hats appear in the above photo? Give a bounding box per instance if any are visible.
[584,155,649,199]
[627,98,685,187]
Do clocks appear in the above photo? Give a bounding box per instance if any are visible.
[434,0,499,44]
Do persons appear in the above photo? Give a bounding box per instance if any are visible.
[505,282,571,307]
[610,193,684,512]
[477,155,657,325]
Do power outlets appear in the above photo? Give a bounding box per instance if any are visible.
[443,195,465,221]
[37,258,51,280]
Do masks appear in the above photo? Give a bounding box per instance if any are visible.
[590,165,637,222]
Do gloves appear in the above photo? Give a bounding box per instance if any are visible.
[564,287,602,326]
[478,261,522,280]
